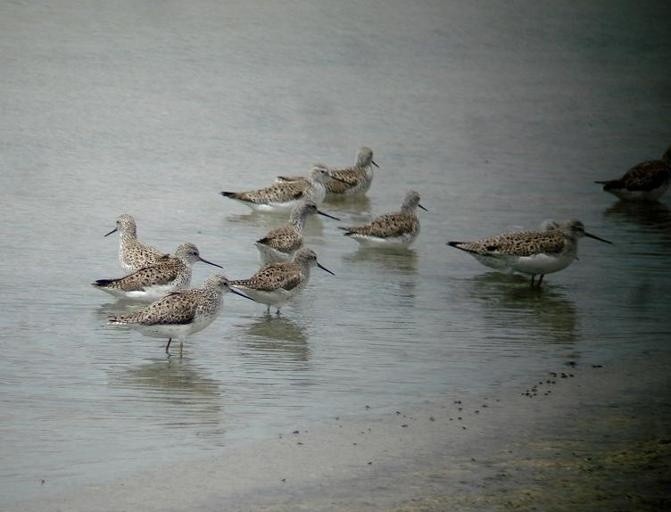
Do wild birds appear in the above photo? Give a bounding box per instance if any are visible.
[444,217,560,271]
[106,214,171,269]
[592,145,670,202]
[253,199,337,260]
[275,146,380,198]
[105,274,255,355]
[91,243,224,304]
[474,218,614,284]
[217,247,335,314]
[338,189,427,251]
[221,161,352,212]
